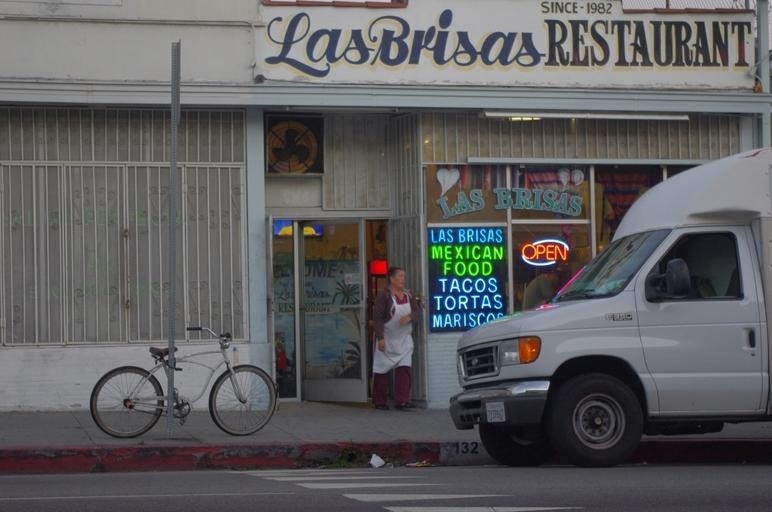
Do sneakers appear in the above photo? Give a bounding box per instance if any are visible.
[376,401,414,410]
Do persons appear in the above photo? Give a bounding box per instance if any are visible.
[522,269,562,310]
[372,266,420,410]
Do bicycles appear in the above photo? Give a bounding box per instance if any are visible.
[89,327,276,439]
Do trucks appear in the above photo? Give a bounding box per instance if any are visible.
[451,147,770,467]
